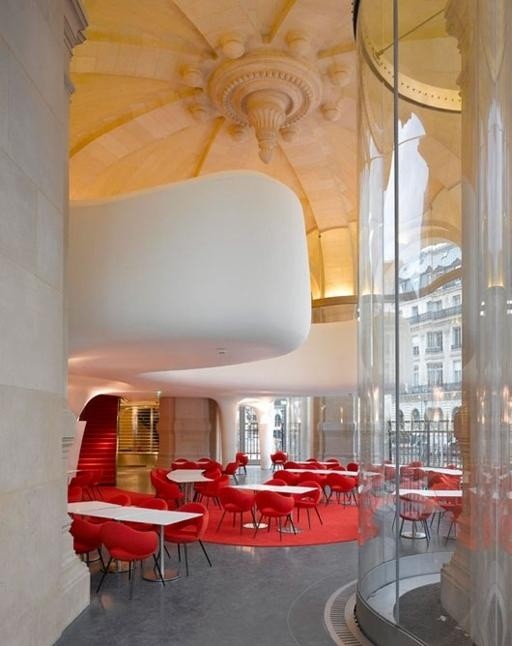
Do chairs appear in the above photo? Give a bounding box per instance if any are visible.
[101,494,168,511]
[148,451,465,550]
[97,521,166,598]
[72,517,111,572]
[156,501,212,575]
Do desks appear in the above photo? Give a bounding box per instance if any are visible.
[68,500,121,561]
[117,510,203,582]
[77,506,141,572]
